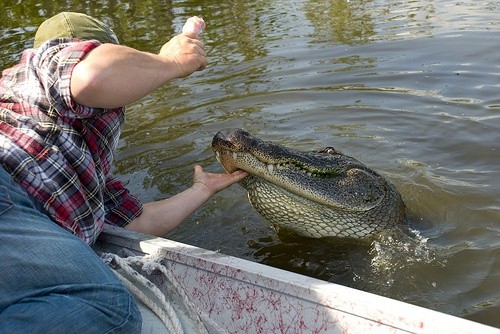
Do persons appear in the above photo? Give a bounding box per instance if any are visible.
[0,12,251,334]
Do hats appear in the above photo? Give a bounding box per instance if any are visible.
[33,11,120,48]
